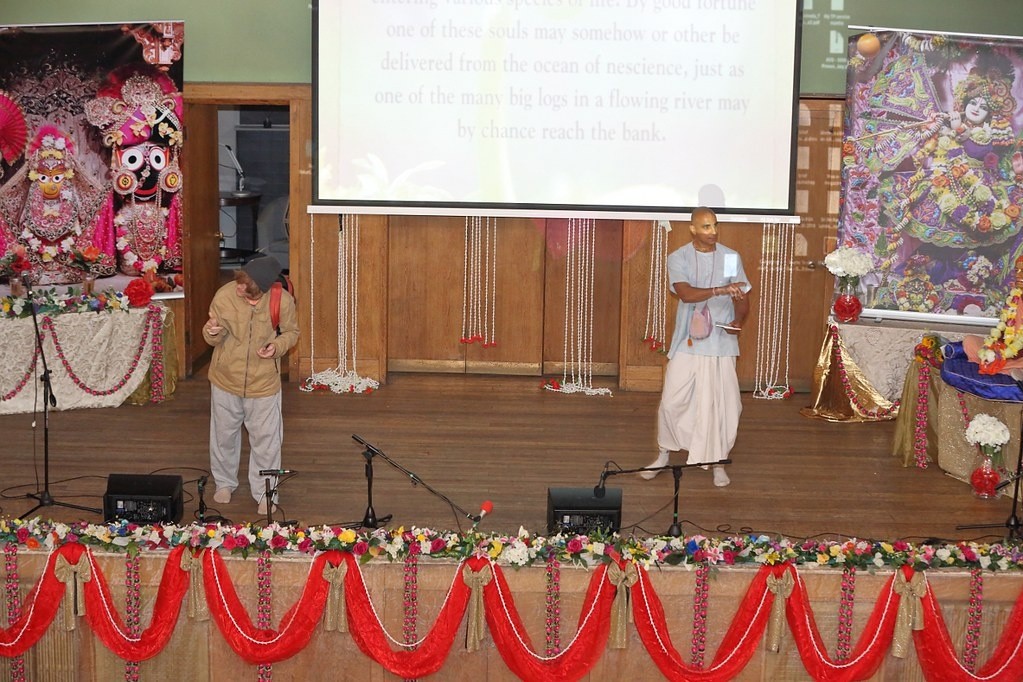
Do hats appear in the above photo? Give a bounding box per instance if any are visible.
[243,257,283,294]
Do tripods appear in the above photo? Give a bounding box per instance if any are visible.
[340,434,476,529]
[18,295,104,523]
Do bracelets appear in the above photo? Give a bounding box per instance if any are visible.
[713,288,715,295]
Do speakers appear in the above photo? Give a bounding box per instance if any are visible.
[103,474,183,526]
[546,486,622,536]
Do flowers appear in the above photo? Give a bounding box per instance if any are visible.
[824,246,873,277]
[915,332,953,366]
[966,412,1010,447]
[0,515,1023,573]
[0,227,154,320]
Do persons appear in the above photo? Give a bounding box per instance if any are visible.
[202,255,300,515]
[640,206,753,487]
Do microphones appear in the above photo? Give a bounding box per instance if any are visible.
[594,463,609,498]
[259,470,294,475]
[21,271,33,296]
[472,500,493,528]
[198,476,208,488]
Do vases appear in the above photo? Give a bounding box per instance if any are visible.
[970,445,1000,499]
[835,275,861,321]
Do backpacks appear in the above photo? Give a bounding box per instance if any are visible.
[269,273,295,331]
